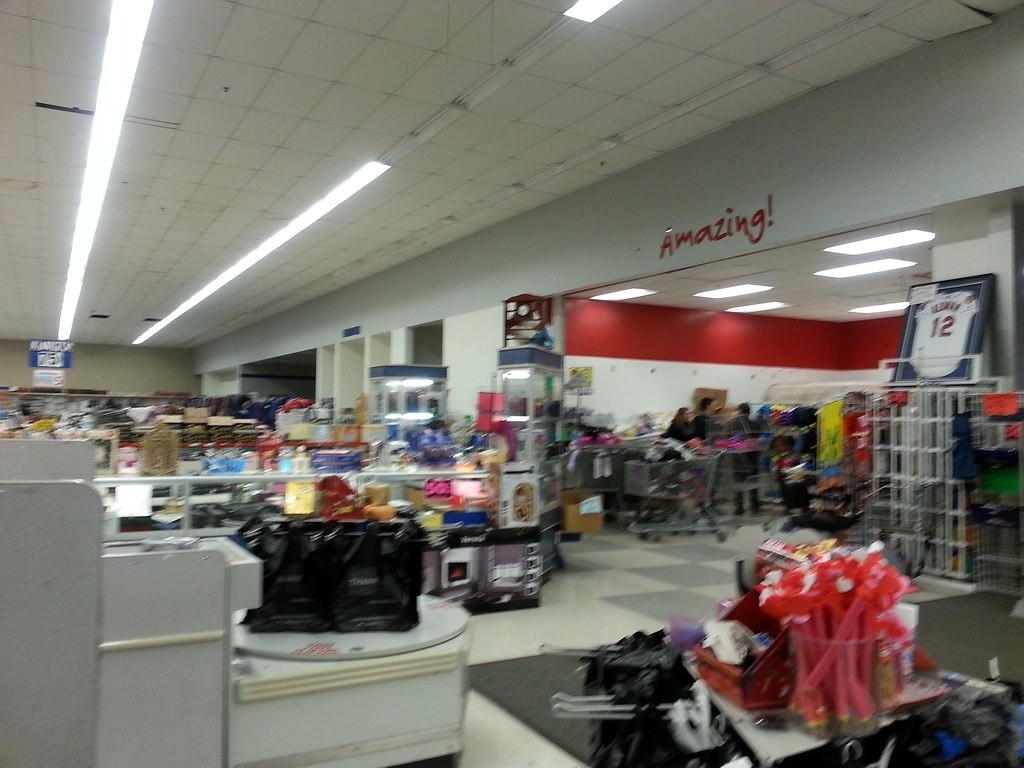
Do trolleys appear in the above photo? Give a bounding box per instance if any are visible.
[623,445,728,544]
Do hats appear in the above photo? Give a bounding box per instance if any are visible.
[735,402,750,410]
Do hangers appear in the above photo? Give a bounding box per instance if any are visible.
[754,389,881,415]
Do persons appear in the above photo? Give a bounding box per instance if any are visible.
[659,407,690,460]
[690,397,715,445]
[721,403,762,515]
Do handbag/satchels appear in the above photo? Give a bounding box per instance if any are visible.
[237,514,428,630]
